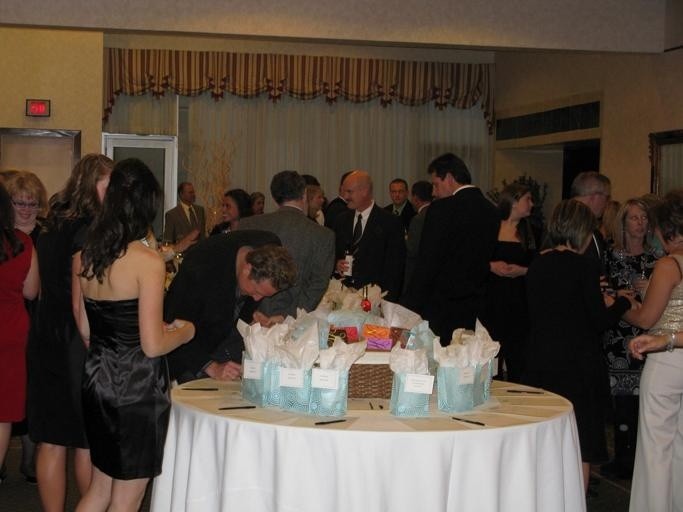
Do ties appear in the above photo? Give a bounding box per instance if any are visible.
[393,210,398,215]
[351,214,362,278]
[188,207,197,227]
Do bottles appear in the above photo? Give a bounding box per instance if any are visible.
[342,248,353,277]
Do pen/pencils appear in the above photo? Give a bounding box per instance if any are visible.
[181,388,219,391]
[452,417,485,426]
[219,406,256,410]
[224,348,242,380]
[315,419,345,424]
[507,390,543,394]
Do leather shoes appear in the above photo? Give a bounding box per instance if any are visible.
[600,458,633,479]
[18,464,37,485]
[587,477,601,499]
[0,465,7,480]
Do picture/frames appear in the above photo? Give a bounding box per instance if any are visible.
[647,128,683,200]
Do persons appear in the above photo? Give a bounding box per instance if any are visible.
[2,144,683,510]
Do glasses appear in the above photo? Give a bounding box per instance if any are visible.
[595,191,611,201]
[9,198,39,209]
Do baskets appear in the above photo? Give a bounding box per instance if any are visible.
[348,364,394,400]
